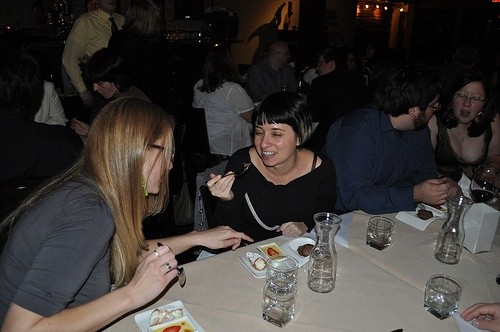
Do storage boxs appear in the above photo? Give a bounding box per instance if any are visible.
[457,202,500,255]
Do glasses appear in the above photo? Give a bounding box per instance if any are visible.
[428,102,442,112]
[454,91,485,103]
[147,141,176,158]
[317,59,324,67]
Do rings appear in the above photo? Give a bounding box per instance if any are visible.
[165,262,172,271]
[152,249,159,258]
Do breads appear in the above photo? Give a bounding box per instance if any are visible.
[150,309,184,327]
[253,257,267,271]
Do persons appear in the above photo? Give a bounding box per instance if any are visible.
[0,95,255,332]
[322,60,469,217]
[436,18,500,113]
[428,71,500,199]
[61,0,170,142]
[192,8,374,165]
[207,92,334,254]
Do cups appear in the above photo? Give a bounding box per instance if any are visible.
[469,162,500,204]
[48,73,56,82]
[366,216,395,251]
[280,81,289,91]
[424,274,462,320]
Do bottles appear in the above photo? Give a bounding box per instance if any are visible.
[307,212,342,293]
[296,80,304,98]
[46,0,77,50]
[434,194,473,264]
[262,255,298,328]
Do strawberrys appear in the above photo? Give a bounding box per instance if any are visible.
[163,325,181,332]
[267,248,279,256]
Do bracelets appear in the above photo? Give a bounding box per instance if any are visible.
[487,163,500,173]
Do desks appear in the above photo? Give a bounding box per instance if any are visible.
[95,232,465,332]
[313,202,500,319]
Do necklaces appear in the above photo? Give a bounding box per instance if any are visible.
[452,115,474,143]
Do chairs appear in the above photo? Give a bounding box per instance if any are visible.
[143,104,230,232]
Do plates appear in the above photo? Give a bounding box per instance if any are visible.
[237,236,316,279]
[135,301,206,332]
[419,203,448,218]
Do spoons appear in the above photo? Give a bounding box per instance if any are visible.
[156,242,187,287]
[221,164,250,179]
